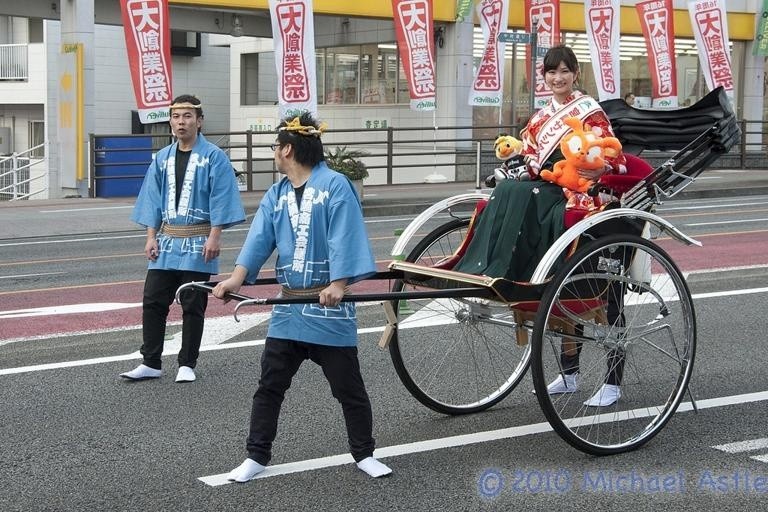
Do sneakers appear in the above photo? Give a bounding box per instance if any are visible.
[584,386,624,408]
[174,365,196,383]
[532,373,578,395]
[228,458,264,482]
[358,455,392,478]
[120,364,163,379]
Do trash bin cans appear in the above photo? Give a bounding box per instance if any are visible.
[94,136,152,197]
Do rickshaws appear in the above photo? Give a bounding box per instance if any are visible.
[174,86,743,457]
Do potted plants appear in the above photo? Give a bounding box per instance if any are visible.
[323,144,370,202]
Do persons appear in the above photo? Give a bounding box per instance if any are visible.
[623,93,637,106]
[527,219,654,408]
[116,92,246,383]
[443,47,630,282]
[208,106,398,483]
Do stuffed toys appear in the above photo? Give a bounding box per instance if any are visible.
[489,131,538,184]
[539,115,624,194]
